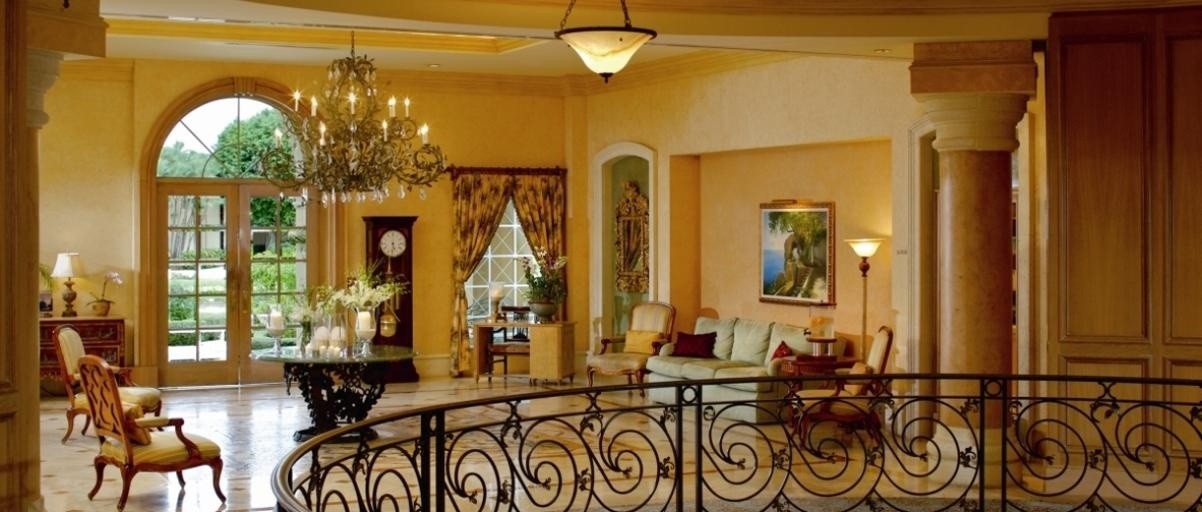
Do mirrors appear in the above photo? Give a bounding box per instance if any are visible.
[614,180,649,293]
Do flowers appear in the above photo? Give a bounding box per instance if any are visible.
[286,285,316,322]
[521,246,569,301]
[83,271,124,306]
[315,265,410,312]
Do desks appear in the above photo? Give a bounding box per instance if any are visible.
[474,320,579,387]
[248,345,420,445]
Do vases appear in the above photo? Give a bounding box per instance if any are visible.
[91,302,110,317]
[299,321,311,352]
[527,300,558,324]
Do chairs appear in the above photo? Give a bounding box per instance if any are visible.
[77,355,226,512]
[791,325,893,450]
[52,323,164,445]
[585,302,676,398]
[486,306,531,383]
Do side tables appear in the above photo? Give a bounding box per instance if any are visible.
[780,356,862,428]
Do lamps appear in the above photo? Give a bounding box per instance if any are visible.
[554,0,657,84]
[486,281,506,324]
[843,238,885,361]
[49,253,88,317]
[263,30,448,208]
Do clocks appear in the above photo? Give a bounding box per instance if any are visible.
[360,215,420,385]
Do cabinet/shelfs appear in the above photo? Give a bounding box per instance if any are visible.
[39,318,125,398]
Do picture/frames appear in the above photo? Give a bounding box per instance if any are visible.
[758,199,837,307]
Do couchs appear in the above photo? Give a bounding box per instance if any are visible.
[646,317,814,424]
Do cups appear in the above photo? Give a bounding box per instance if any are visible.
[332,312,351,364]
[313,312,334,365]
[267,304,290,356]
[354,306,376,355]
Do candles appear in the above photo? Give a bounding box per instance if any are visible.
[358,311,371,329]
[270,309,282,329]
[331,326,345,340]
[315,327,329,340]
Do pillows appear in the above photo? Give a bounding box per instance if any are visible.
[770,341,796,361]
[624,330,659,354]
[667,331,718,359]
[844,362,873,396]
